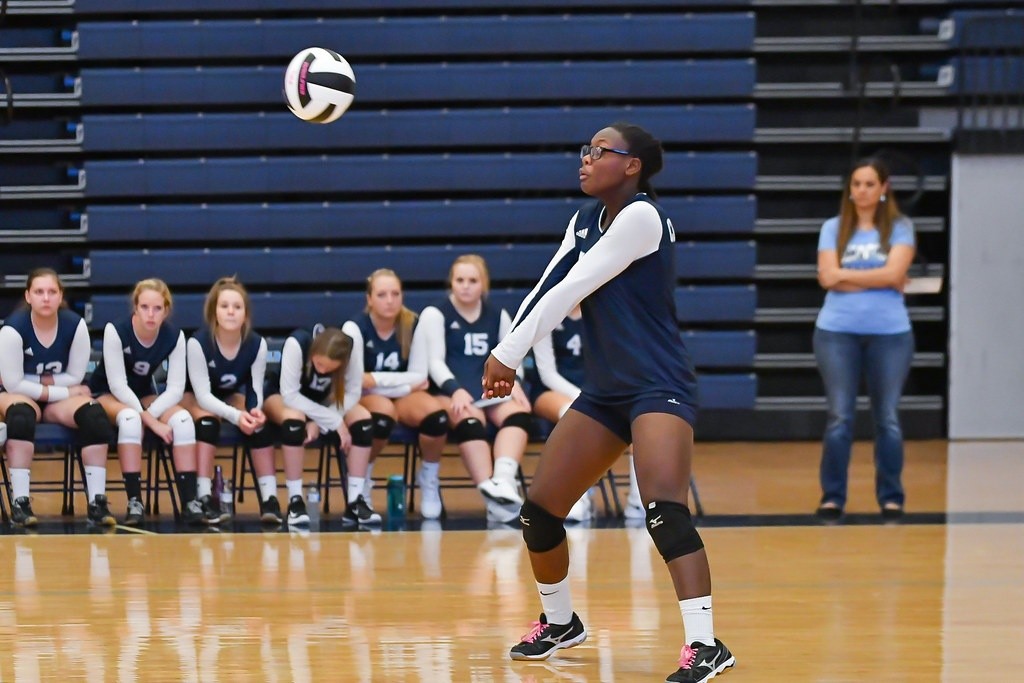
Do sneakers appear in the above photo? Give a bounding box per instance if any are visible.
[510,612,587,661]
[665,638,735,683]
[342,494,383,524]
[86,494,117,526]
[286,495,311,525]
[9,497,38,526]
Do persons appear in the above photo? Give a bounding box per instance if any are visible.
[263,320,382,525]
[178,277,283,523]
[813,158,916,520]
[482,123,735,683]
[340,269,450,519]
[529,304,646,522]
[89,279,219,525]
[421,254,533,522]
[0,268,118,526]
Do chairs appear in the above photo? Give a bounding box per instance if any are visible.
[0,339,707,526]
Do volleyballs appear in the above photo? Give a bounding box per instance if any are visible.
[281,47,358,123]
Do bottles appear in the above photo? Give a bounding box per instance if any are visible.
[306,487,320,524]
[387,473,406,521]
[587,484,596,519]
[212,465,233,520]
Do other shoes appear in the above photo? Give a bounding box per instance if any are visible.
[478,475,523,512]
[194,495,220,523]
[260,496,283,524]
[418,470,443,520]
[816,500,842,521]
[625,498,647,519]
[484,501,522,523]
[178,511,220,533]
[564,493,596,520]
[883,502,903,521]
[123,496,143,525]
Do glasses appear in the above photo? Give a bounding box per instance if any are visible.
[580,145,646,161]
